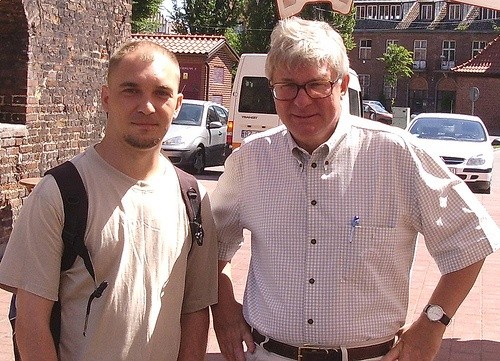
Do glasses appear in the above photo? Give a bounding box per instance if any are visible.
[268,75,342,101]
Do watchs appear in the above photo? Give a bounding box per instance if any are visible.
[423,303,450,325]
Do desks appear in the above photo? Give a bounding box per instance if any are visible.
[19,178,41,194]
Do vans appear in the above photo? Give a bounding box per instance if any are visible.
[226,54,365,154]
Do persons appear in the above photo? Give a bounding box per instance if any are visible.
[1,41,218,361]
[208,17,499,361]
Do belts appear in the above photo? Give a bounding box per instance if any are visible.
[249,324,395,361]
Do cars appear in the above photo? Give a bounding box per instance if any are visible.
[159,98,229,175]
[406,112,500,194]
[361,100,393,125]
